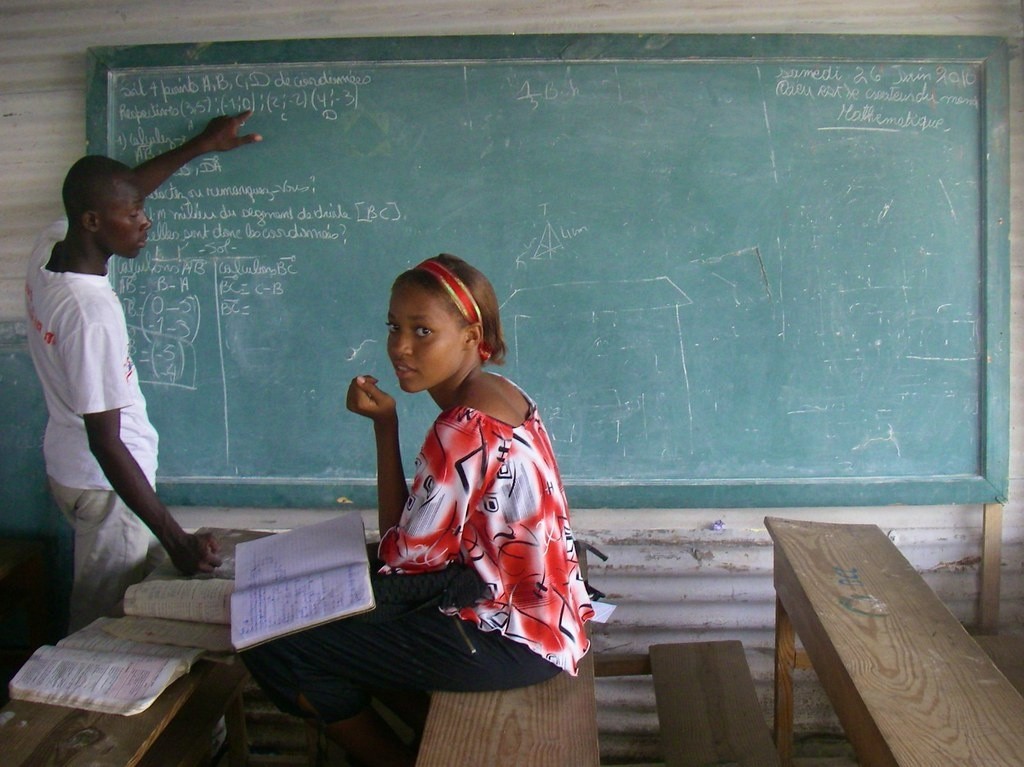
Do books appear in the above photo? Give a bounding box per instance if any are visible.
[9,617,205,715]
[229,511,377,652]
[99,555,238,651]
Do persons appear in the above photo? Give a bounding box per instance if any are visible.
[25,108,264,634]
[237,252,594,767]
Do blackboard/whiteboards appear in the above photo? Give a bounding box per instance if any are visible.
[78,26,1013,515]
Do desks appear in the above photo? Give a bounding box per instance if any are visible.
[414,541,600,767]
[0,526,304,766]
[763,515,1024,764]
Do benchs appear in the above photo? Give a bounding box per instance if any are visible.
[592,640,786,767]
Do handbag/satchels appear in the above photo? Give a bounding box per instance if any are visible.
[358,548,491,652]
